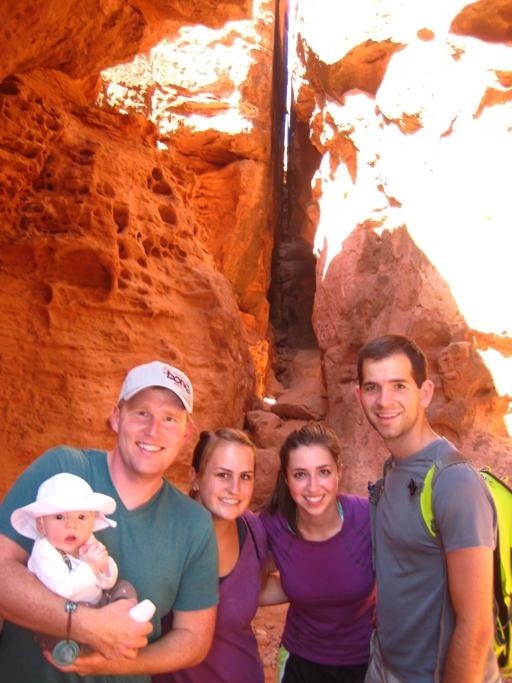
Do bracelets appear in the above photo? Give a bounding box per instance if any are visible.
[63,601,78,643]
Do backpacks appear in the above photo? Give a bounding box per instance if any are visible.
[420,454,512,673]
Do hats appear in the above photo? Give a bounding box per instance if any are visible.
[10,472,118,538]
[117,362,194,413]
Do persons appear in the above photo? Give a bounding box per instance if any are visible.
[152,427,291,683]
[10,473,159,661]
[255,422,374,683]
[0,359,220,682]
[361,334,504,682]
